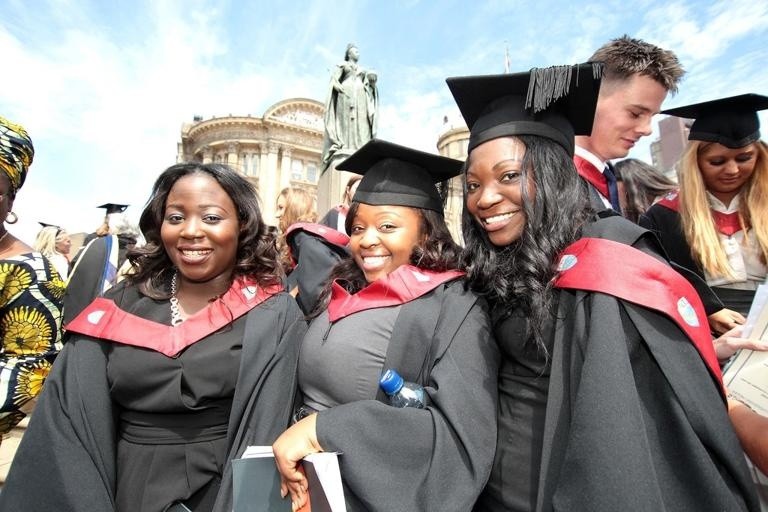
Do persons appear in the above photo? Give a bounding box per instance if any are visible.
[317,175,362,236]
[269,139,503,510]
[572,33,768,362]
[633,86,768,339]
[718,381,768,480]
[318,43,379,169]
[446,60,761,510]
[270,188,316,300]
[1,118,73,446]
[605,154,680,226]
[34,200,140,329]
[1,159,311,511]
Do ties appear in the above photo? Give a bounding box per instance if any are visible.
[603,165,623,214]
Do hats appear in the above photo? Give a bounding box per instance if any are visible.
[660,91,768,150]
[334,137,466,227]
[445,60,604,161]
[97,202,128,213]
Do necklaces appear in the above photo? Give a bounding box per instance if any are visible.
[0,230,8,244]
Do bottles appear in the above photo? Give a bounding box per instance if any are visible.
[379,368,434,411]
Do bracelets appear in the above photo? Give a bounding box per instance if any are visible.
[725,393,740,401]
